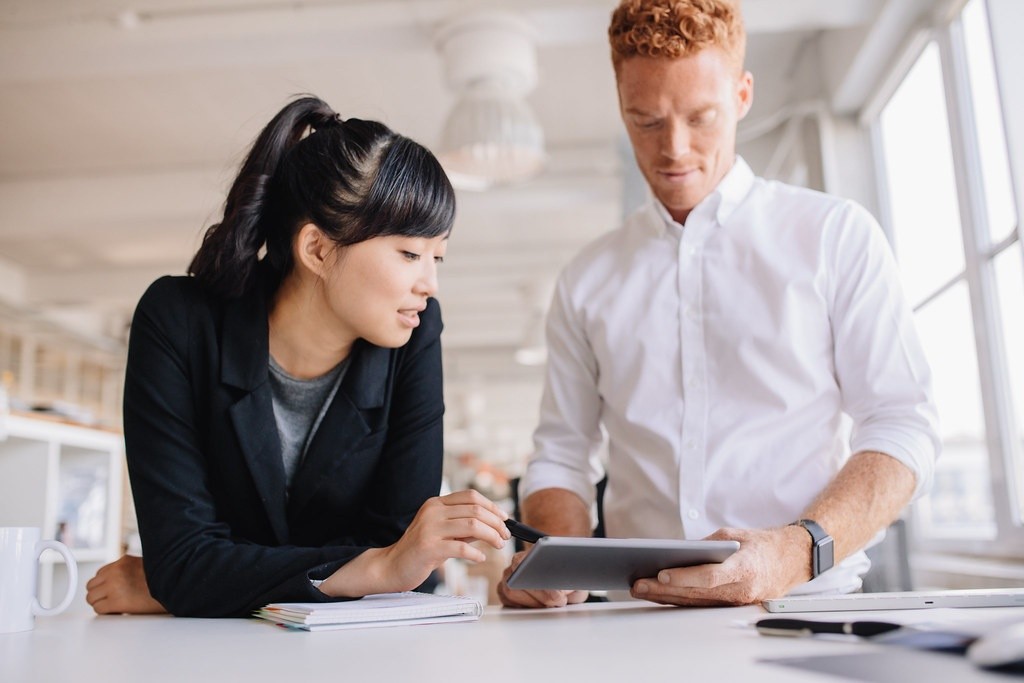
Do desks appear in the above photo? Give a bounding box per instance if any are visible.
[1,600,1024,683]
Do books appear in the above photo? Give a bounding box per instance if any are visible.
[253,591,484,631]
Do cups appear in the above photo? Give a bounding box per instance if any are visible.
[0,526,79,634]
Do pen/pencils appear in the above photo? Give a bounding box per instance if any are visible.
[503,518,547,543]
[748,618,900,638]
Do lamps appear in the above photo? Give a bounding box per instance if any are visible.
[434,24,542,180]
[515,308,549,365]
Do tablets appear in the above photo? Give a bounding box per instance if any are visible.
[507,536,740,589]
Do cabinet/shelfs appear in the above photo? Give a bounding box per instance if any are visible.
[0,413,126,610]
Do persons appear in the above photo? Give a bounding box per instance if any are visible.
[496,0,948,608]
[87,100,511,619]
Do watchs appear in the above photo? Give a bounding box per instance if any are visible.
[790,519,834,580]
